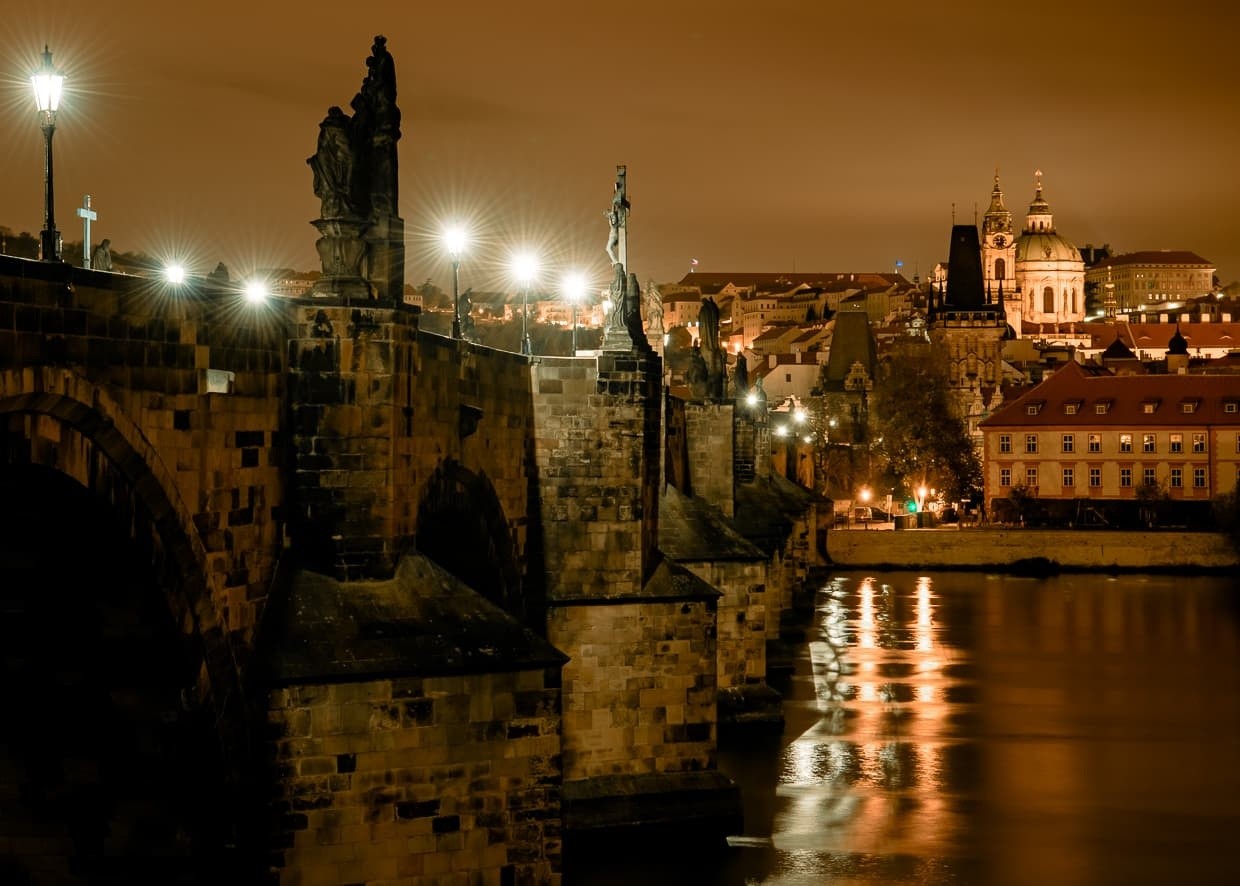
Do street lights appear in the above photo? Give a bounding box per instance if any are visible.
[29,43,68,260]
[861,489,872,530]
[508,246,541,355]
[564,272,583,355]
[440,213,474,342]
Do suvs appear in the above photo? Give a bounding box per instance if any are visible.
[856,507,895,522]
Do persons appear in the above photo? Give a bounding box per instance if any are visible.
[93,239,112,271]
[627,272,641,325]
[697,297,720,350]
[610,265,627,326]
[735,352,747,392]
[306,106,359,222]
[601,200,619,264]
[645,280,665,330]
[349,35,397,119]
